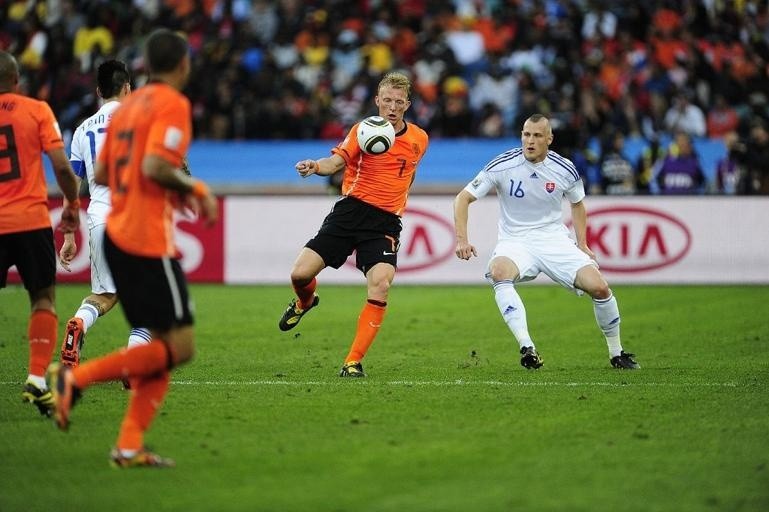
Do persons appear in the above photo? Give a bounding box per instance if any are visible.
[278,70,429,379]
[46,26,219,470]
[1,1,768,195]
[0,51,80,417]
[58,59,154,392]
[453,114,641,369]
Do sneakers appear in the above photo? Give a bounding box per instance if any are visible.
[340,361,365,377]
[59,318,83,367]
[520,345,544,369]
[50,364,80,432]
[122,377,130,389]
[108,448,174,468]
[608,351,641,371]
[279,293,319,331]
[20,380,54,418]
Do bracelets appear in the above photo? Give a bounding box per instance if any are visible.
[63,195,81,210]
[314,160,320,174]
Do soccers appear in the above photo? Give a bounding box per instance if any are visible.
[356,116,395,155]
